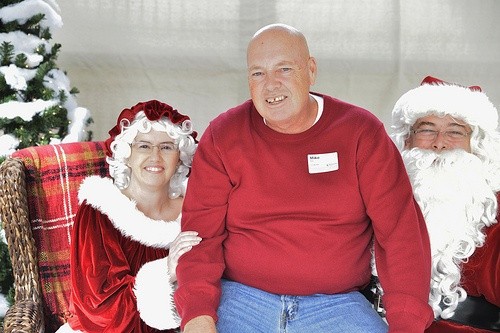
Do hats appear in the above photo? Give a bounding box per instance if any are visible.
[391,76,498,132]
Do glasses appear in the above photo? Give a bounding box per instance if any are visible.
[131,142,180,154]
[411,126,471,141]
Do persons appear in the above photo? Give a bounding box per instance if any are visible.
[369,75,497,332]
[70,100,203,333]
[173,24,434,333]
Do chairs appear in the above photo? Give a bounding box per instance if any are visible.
[0,142,108,333]
